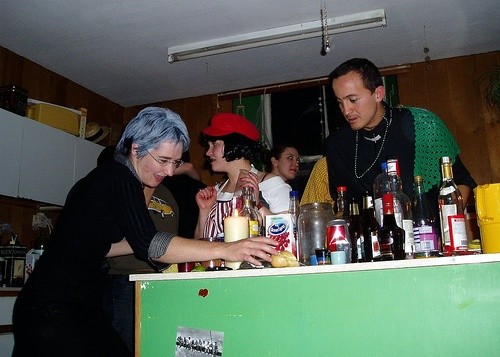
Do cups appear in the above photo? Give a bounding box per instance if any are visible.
[473,182,500,254]
[197,237,221,268]
[178,261,195,273]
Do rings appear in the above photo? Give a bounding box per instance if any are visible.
[200,190,202,191]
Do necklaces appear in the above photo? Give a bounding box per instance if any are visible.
[354,112,388,178]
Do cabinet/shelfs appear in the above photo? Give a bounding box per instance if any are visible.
[0,107,106,206]
[0,290,18,357]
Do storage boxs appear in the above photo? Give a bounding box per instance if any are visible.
[266,214,297,257]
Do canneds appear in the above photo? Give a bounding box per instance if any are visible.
[326,219,352,262]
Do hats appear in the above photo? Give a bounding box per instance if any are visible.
[203,112,260,141]
[84,122,110,142]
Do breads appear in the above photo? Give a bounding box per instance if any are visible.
[271,251,300,267]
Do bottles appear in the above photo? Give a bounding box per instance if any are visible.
[0,226,48,287]
[287,156,467,265]
[238,186,263,238]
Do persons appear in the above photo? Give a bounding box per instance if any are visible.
[12,106,279,357]
[325,58,482,252]
[268,143,299,193]
[194,113,294,240]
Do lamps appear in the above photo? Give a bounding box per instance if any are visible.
[167,9,386,63]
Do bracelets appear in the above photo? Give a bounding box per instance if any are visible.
[256,200,264,211]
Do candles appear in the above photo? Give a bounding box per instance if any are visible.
[224,209,248,270]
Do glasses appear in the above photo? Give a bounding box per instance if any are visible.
[144,146,183,169]
